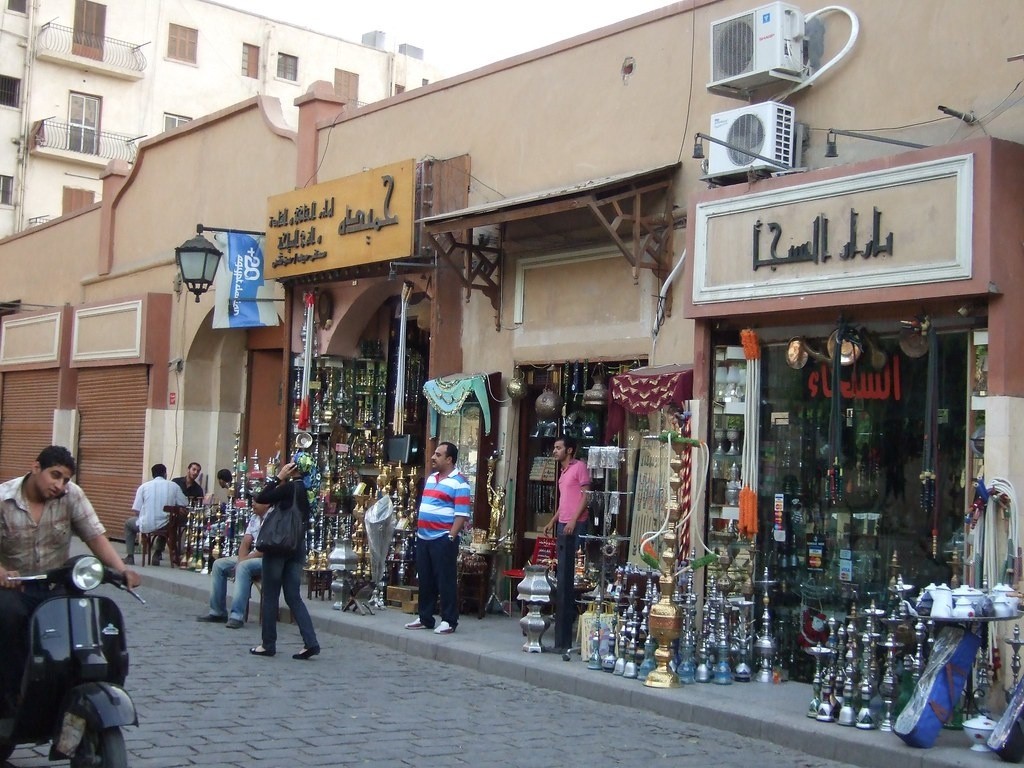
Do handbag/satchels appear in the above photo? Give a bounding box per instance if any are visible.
[256,480,305,554]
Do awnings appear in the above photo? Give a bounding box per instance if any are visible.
[415,162,681,311]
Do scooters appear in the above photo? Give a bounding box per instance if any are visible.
[0,554,146,768]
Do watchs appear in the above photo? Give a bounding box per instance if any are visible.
[448,535,454,542]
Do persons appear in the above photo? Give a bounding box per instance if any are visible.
[405,441,471,633]
[0,445,142,720]
[121,464,189,565]
[196,497,275,628]
[250,452,320,659]
[153,462,204,562]
[544,436,590,618]
[217,469,232,489]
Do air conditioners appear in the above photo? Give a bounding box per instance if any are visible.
[707,101,794,187]
[709,1,805,94]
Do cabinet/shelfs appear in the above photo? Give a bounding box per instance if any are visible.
[707,345,756,544]
[520,367,562,541]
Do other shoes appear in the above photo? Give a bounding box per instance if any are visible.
[151,554,163,565]
[250,646,276,656]
[122,554,134,565]
[292,644,320,659]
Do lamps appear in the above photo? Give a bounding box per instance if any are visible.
[916,315,933,335]
[175,224,265,304]
[956,298,989,318]
[693,133,796,169]
[388,262,436,286]
[826,129,930,158]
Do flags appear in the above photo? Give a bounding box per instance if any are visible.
[213,232,279,327]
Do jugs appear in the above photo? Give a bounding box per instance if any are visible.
[919,580,1018,618]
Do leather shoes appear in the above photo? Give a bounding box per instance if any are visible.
[434,621,453,634]
[196,613,228,623]
[226,618,244,629]
[405,617,427,629]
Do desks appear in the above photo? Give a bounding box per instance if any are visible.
[458,572,487,621]
[906,612,1024,727]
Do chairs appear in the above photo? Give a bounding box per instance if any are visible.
[141,505,185,569]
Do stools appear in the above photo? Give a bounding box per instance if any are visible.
[308,569,333,602]
[503,568,528,618]
[342,582,377,615]
